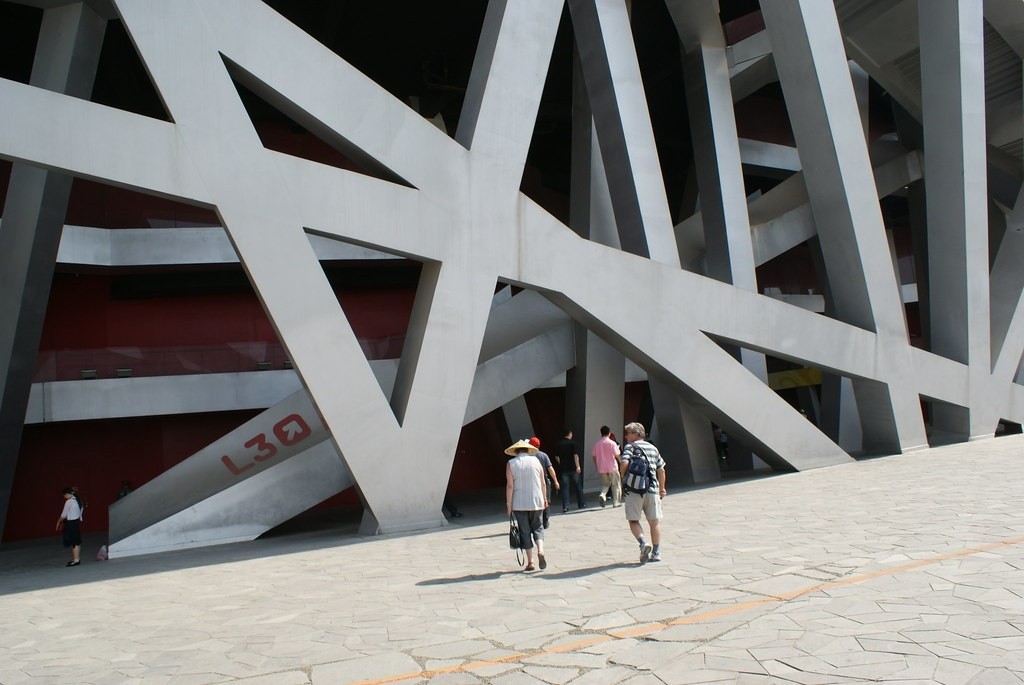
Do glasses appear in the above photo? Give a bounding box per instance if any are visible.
[623,431,634,436]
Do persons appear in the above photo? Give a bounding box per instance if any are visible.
[529,437,560,529]
[55,487,83,567]
[637,404,808,492]
[620,422,667,563]
[592,425,622,507]
[554,429,588,512]
[504,438,548,574]
[606,432,621,500]
[117,480,133,500]
[445,488,465,518]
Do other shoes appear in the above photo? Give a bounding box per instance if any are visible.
[578,503,589,508]
[538,552,546,569]
[563,508,569,512]
[613,503,622,508]
[66,560,80,567]
[451,512,464,518]
[599,494,606,508]
[522,564,535,573]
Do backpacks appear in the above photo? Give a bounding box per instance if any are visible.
[623,441,652,494]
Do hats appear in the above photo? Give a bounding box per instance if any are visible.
[505,438,539,456]
[530,437,540,447]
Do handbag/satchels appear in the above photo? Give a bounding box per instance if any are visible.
[510,511,521,549]
[96,544,108,560]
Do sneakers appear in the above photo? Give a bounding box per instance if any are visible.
[652,550,660,561]
[640,543,651,563]
